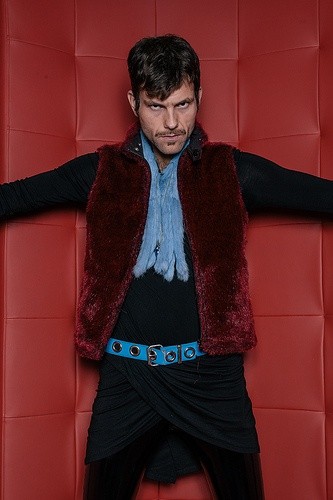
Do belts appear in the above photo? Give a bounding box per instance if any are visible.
[111,340,200,370]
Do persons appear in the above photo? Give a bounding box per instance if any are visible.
[1,33,333,500]
